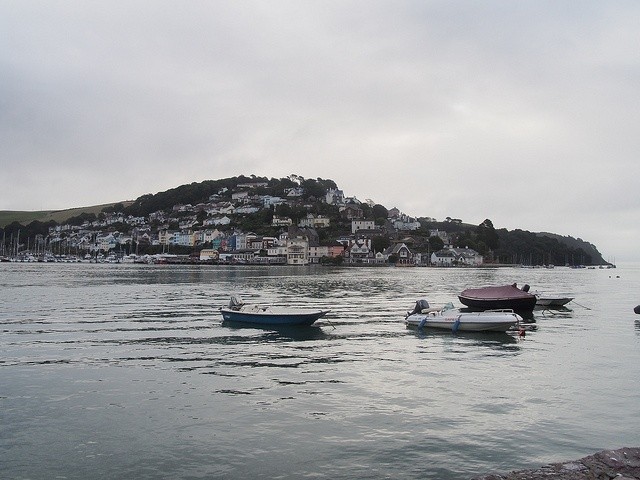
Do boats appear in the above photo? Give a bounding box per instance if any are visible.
[458,282,537,313]
[218,295,333,326]
[536,296,575,308]
[405,306,523,333]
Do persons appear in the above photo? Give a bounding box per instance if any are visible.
[407,300,421,316]
[523,284,530,292]
